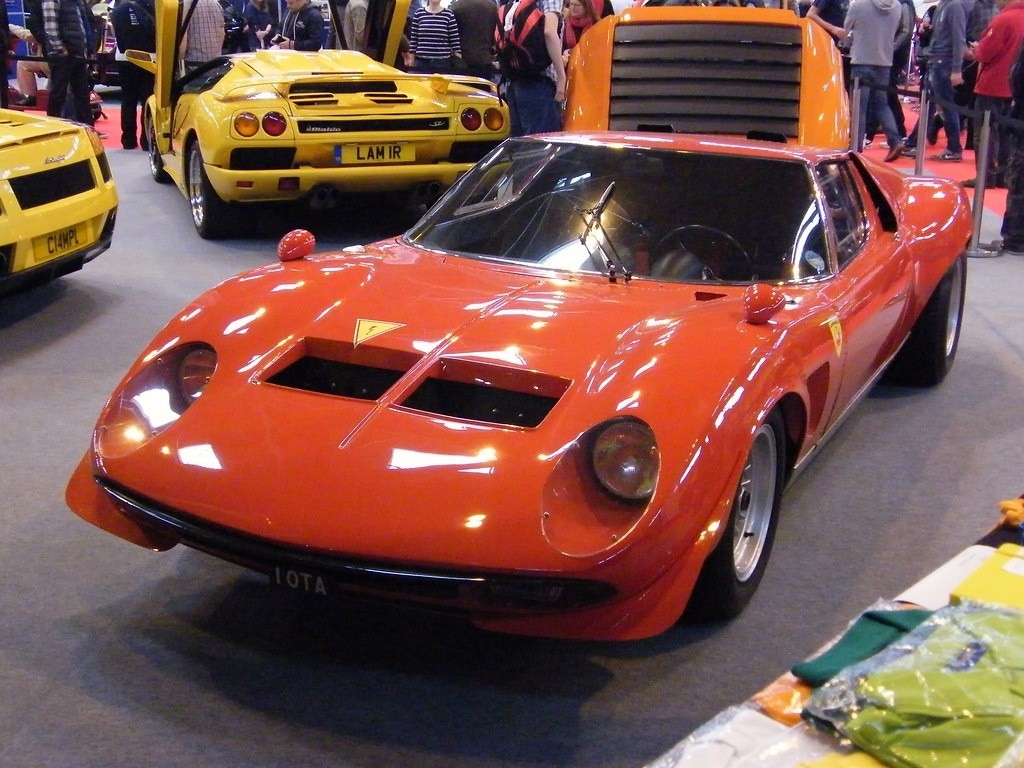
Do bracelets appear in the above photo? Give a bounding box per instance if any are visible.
[289,40,291,49]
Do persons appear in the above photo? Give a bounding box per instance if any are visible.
[409,0,462,73]
[0,0,108,139]
[805,0,1024,255]
[111,0,630,150]
[633,0,812,17]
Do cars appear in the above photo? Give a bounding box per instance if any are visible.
[222,12,239,43]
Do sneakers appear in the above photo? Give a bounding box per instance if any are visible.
[900,146,918,157]
[930,148,962,162]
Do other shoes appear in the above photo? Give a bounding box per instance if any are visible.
[960,172,995,189]
[123,143,138,149]
[927,131,937,145]
[8,94,36,108]
[96,132,108,138]
[991,240,1024,255]
[879,142,890,149]
[884,142,905,162]
[863,139,873,149]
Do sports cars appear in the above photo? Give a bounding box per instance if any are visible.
[123,0,512,238]
[86,21,123,92]
[0,107,120,299]
[564,5,853,155]
[61,129,973,643]
